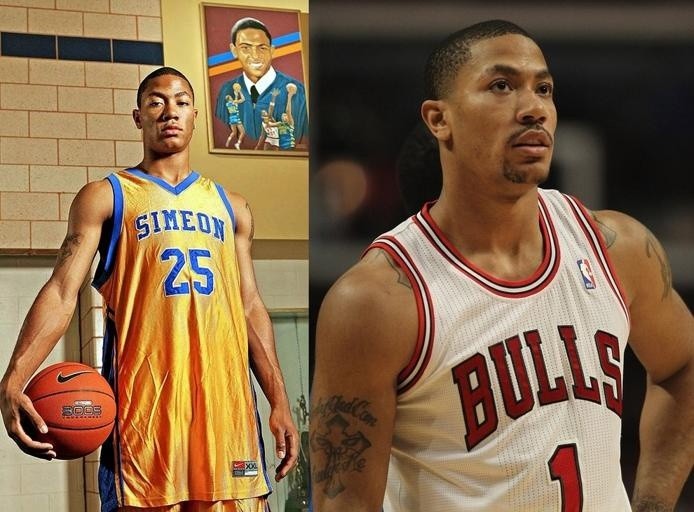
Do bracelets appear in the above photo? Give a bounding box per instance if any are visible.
[269,102,275,106]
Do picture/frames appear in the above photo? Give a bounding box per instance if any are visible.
[197,0,309,157]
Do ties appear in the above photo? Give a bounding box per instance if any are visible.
[250,86,260,109]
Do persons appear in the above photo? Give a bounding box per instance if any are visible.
[267,87,295,150]
[307,20,692,510]
[0,67,301,511]
[224,90,245,151]
[212,17,308,148]
[254,110,282,152]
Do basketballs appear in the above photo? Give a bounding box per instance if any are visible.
[287,83,297,93]
[20,362,118,460]
[233,83,240,91]
